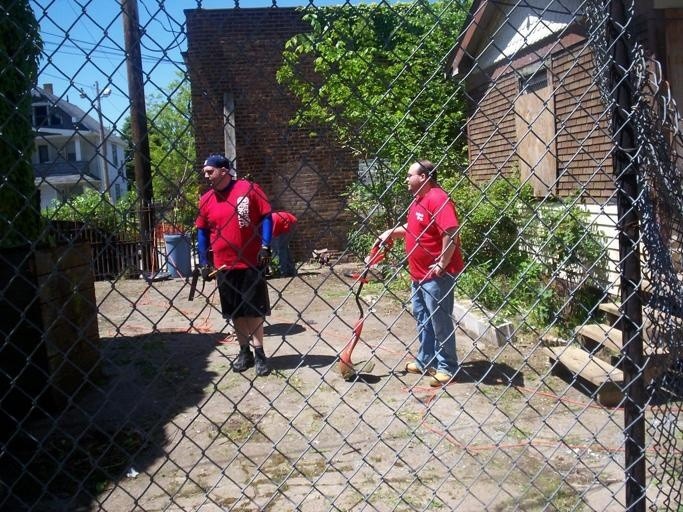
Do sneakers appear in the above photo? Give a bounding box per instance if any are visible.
[231,350,255,372]
[430,369,460,387]
[254,354,271,375]
[403,360,436,374]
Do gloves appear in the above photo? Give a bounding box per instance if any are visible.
[256,247,272,269]
[200,269,218,283]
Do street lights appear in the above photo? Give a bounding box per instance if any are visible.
[81,81,112,201]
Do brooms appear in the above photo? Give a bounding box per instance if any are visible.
[148,228,156,285]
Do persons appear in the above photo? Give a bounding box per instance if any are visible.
[372,161,463,387]
[195,156,299,376]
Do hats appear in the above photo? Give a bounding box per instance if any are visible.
[202,154,236,168]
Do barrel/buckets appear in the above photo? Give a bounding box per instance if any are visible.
[164,235,191,277]
